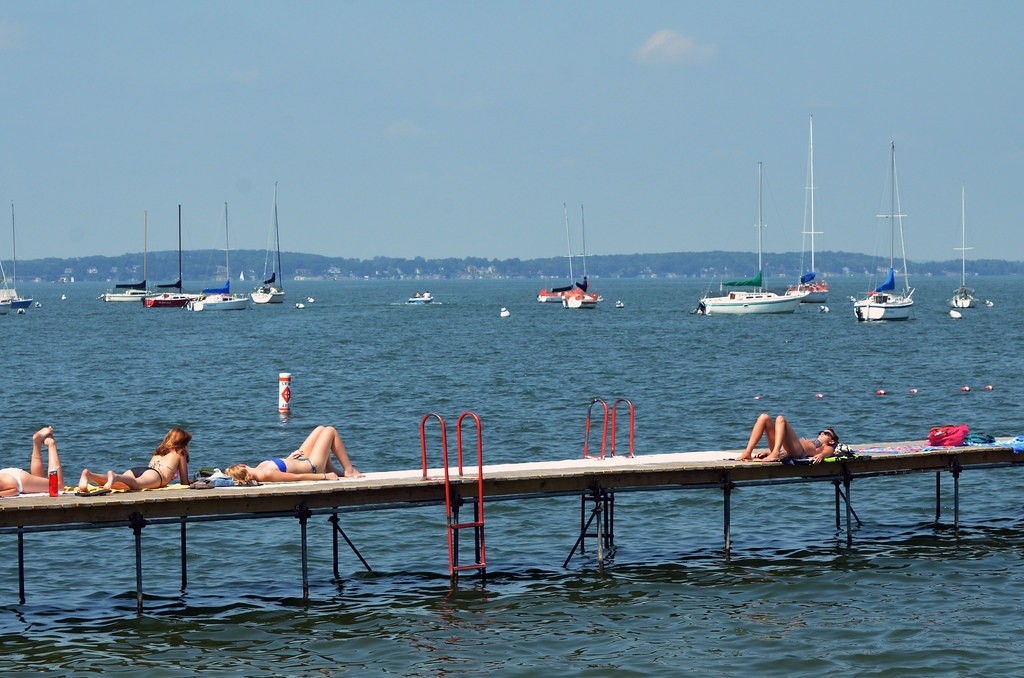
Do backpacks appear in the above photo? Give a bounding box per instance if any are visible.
[928,424,970,447]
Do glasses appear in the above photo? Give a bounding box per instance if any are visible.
[818,431,833,438]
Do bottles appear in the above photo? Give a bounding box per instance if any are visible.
[49,470,58,497]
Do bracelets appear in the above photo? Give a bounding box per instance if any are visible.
[324,472,326,479]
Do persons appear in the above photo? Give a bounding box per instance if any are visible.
[0,427,65,496]
[736,413,838,464]
[78,429,192,490]
[225,425,366,480]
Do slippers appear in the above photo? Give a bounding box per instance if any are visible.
[230,478,263,487]
[190,479,214,489]
[80,489,113,497]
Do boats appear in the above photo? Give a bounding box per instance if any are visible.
[407,296,434,303]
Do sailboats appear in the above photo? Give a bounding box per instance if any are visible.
[695,114,979,320]
[536,198,604,310]
[0,202,34,316]
[100,178,287,311]
